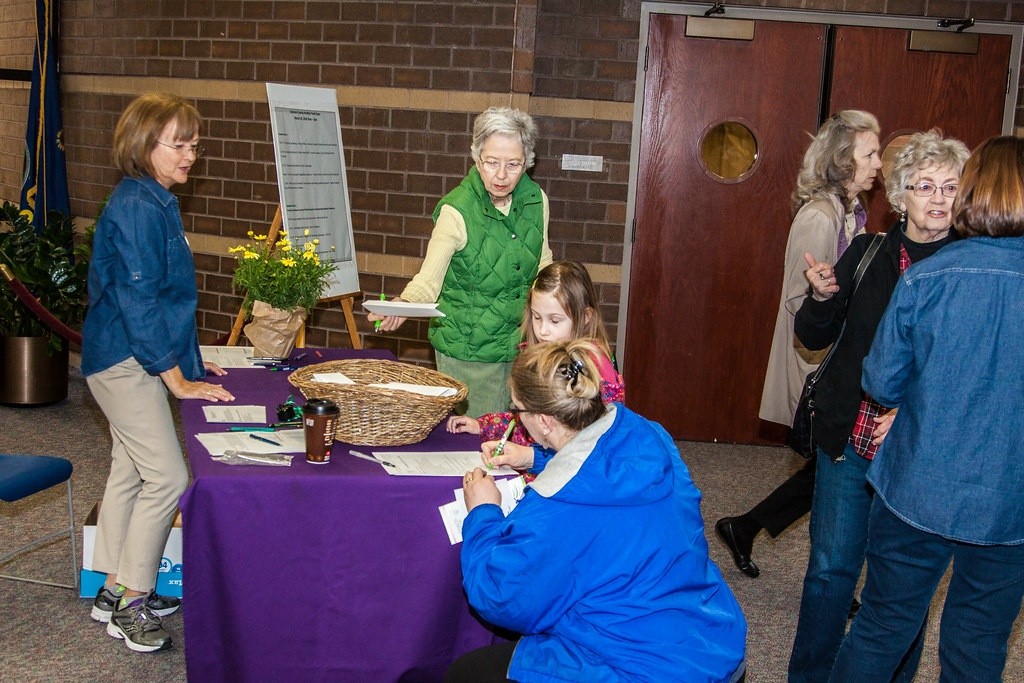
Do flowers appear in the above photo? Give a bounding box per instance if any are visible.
[228,228,341,321]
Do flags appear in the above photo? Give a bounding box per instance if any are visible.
[20,0,75,266]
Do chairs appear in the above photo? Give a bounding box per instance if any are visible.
[0,454,78,590]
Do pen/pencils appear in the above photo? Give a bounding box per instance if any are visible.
[294,353,306,361]
[315,350,323,358]
[488,418,516,471]
[349,450,395,468]
[246,356,283,364]
[249,433,280,446]
[373,293,386,333]
[268,421,303,428]
[226,426,275,432]
[270,365,302,371]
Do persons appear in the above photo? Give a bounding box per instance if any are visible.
[367,106,553,418]
[446,260,625,484]
[714,109,1024,683]
[461,337,748,683]
[81,93,236,652]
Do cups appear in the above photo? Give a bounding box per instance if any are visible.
[301,398,340,464]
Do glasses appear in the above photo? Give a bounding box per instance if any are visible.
[904,181,959,199]
[152,138,206,156]
[474,154,529,175]
[508,400,537,413]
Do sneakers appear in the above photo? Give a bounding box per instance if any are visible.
[89,586,181,652]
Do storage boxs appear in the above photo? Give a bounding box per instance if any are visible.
[80,500,183,598]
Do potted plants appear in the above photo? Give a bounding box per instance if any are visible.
[0,199,88,404]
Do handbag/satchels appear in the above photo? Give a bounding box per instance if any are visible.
[787,369,826,462]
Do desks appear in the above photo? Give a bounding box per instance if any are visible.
[180,349,521,683]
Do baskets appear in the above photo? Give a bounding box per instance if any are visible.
[287,358,470,448]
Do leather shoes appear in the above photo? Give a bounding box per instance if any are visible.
[713,516,761,578]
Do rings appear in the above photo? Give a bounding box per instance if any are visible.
[208,390,211,395]
[819,272,825,280]
[827,278,831,285]
[465,477,472,483]
